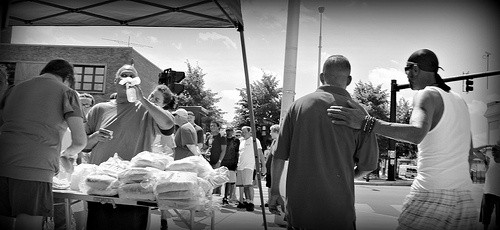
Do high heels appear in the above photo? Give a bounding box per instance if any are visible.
[222,197,229,205]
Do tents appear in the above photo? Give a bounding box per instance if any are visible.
[0,0,268,230]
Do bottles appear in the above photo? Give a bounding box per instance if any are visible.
[123,77,139,105]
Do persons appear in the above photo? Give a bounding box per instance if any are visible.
[0,59,87,230]
[172,108,282,212]
[83,64,176,230]
[327,48,475,230]
[80,94,94,115]
[147,83,175,109]
[268,55,378,230]
[475,143,500,230]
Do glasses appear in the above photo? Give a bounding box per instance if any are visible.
[226,129,233,131]
[81,103,93,108]
[405,65,418,75]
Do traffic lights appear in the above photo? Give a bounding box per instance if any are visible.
[170,69,186,96]
[466,80,474,92]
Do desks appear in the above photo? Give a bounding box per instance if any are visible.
[53,189,214,230]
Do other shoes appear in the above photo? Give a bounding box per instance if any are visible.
[260,203,269,207]
[245,203,254,211]
[237,202,247,208]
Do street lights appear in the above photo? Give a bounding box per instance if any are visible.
[317,5,325,87]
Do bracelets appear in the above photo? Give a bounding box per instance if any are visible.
[361,115,376,134]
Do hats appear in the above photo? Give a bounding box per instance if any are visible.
[40,59,74,75]
[234,130,242,136]
[171,109,188,118]
